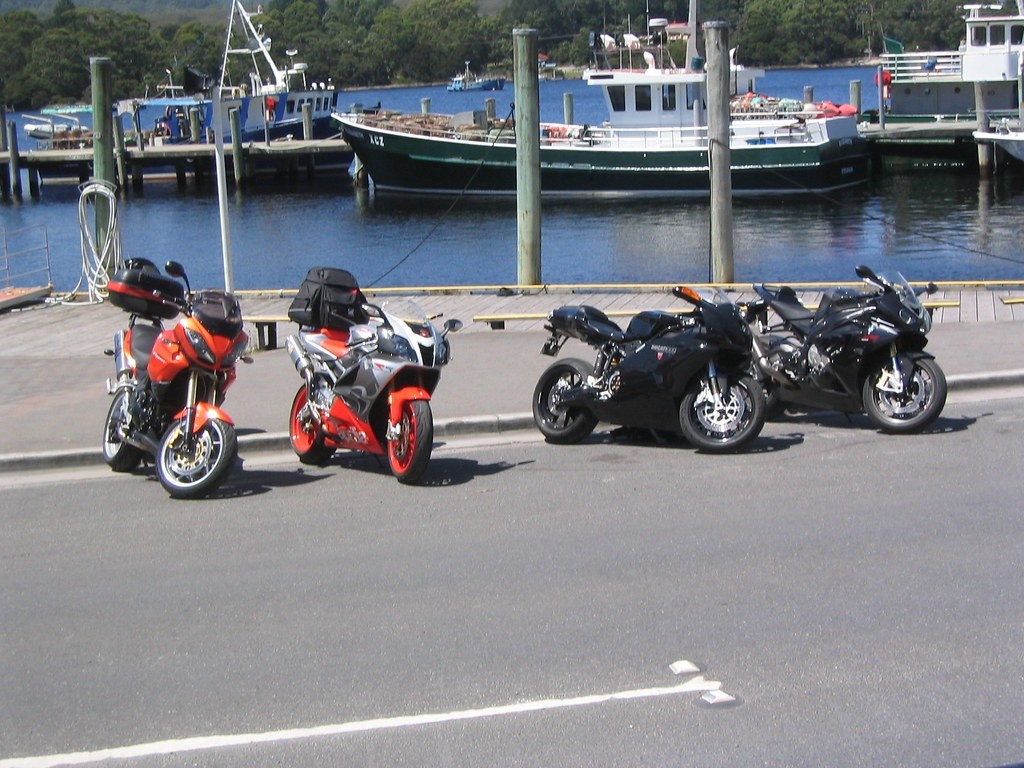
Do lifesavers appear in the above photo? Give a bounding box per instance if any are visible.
[165,106,171,120]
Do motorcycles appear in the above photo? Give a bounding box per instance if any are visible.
[103,259,253,501]
[736,264,947,434]
[532,287,767,455]
[279,291,463,486]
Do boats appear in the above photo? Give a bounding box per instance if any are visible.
[24,124,88,140]
[968,108,1024,164]
[329,0,871,202]
[859,0,1024,175]
[447,61,506,92]
[35,0,355,186]
